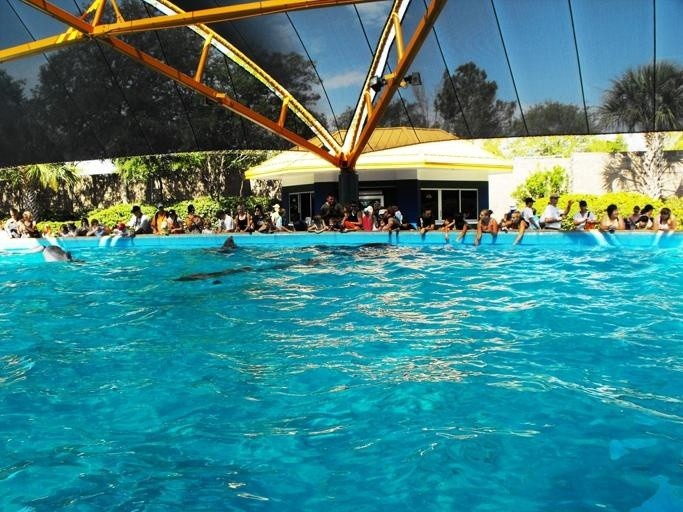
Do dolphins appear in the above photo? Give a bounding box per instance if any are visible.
[218,235,240,254]
[42,244,72,264]
[172,261,316,284]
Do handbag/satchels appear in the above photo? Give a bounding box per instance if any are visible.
[583,211,596,230]
[28,221,40,237]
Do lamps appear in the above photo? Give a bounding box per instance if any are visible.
[403,72,422,88]
[367,72,392,92]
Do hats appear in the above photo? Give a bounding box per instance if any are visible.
[168,209,175,215]
[157,203,164,209]
[550,194,561,199]
[131,205,141,213]
[525,197,536,202]
[378,209,388,215]
[271,203,280,208]
[644,205,656,210]
